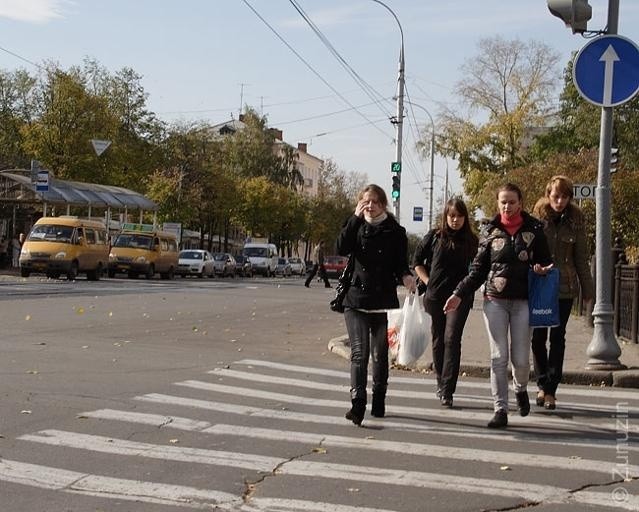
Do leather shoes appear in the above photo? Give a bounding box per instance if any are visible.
[536,389,556,408]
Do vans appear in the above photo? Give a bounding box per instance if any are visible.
[108,229,178,281]
[17,214,111,282]
[241,243,279,278]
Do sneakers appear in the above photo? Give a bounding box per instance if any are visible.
[488,411,507,427]
[516,390,530,416]
[438,387,453,407]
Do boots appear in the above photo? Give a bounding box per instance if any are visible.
[372,389,385,418]
[346,400,365,424]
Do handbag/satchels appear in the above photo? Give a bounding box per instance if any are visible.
[329,254,355,312]
[412,265,429,296]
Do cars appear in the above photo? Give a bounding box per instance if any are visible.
[287,257,306,277]
[210,253,237,278]
[176,249,216,279]
[232,256,252,278]
[275,258,292,278]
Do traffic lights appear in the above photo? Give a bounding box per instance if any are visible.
[390,161,402,173]
[610,146,618,175]
[392,176,400,200]
[547,0,592,34]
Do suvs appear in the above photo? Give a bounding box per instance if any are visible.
[321,256,348,277]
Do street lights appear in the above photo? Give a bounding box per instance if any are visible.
[415,138,449,209]
[393,96,434,232]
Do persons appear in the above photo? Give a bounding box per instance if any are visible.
[305,240,332,288]
[412,199,478,406]
[443,184,551,428]
[527,175,593,408]
[335,185,416,424]
